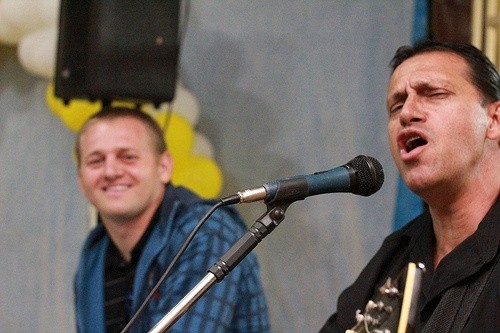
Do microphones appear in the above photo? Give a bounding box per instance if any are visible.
[219,153,385,209]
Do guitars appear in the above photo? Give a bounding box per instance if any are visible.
[339,260,426,333]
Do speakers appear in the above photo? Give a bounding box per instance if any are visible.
[54,0,193,111]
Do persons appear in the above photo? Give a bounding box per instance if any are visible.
[69,107,271,333]
[317,27,500,333]
